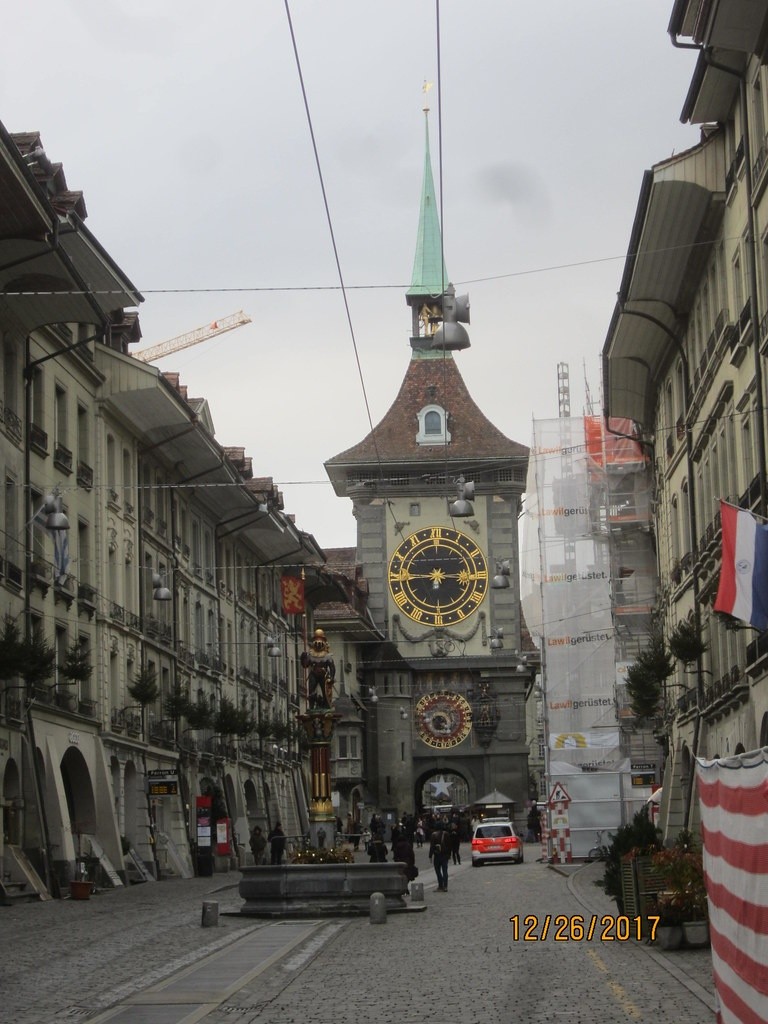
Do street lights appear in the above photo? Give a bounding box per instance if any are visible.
[137,560,172,883]
[24,481,71,899]
[255,634,281,834]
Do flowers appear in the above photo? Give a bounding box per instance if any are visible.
[287,844,353,864]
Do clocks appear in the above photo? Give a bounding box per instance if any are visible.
[387,526,488,627]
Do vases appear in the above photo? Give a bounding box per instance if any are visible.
[239,862,408,911]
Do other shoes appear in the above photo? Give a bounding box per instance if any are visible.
[432,887,447,892]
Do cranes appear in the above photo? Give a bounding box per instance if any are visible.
[128,310,252,362]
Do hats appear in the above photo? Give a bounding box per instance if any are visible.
[276,822,281,827]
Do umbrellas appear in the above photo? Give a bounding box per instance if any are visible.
[474,787,517,804]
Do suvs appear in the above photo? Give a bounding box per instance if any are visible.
[471,817,524,867]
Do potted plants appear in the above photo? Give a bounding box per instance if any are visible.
[594,804,711,951]
[72,841,105,900]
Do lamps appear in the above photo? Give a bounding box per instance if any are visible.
[151,569,173,602]
[489,627,507,650]
[515,655,527,671]
[368,687,379,703]
[489,559,512,590]
[429,283,471,350]
[449,473,476,518]
[399,706,409,720]
[267,634,283,658]
[44,487,72,531]
[535,682,542,698]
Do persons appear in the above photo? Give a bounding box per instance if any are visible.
[317,827,326,848]
[420,303,433,336]
[527,800,543,843]
[300,636,336,709]
[367,808,483,896]
[429,823,452,892]
[335,815,343,834]
[362,828,372,851]
[267,821,286,866]
[347,813,353,843]
[354,817,363,851]
[249,825,267,866]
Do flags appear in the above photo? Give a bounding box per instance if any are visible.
[690,746,768,1024]
[281,576,304,614]
[52,529,72,584]
[713,501,768,632]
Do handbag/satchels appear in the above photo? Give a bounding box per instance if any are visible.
[434,844,441,853]
